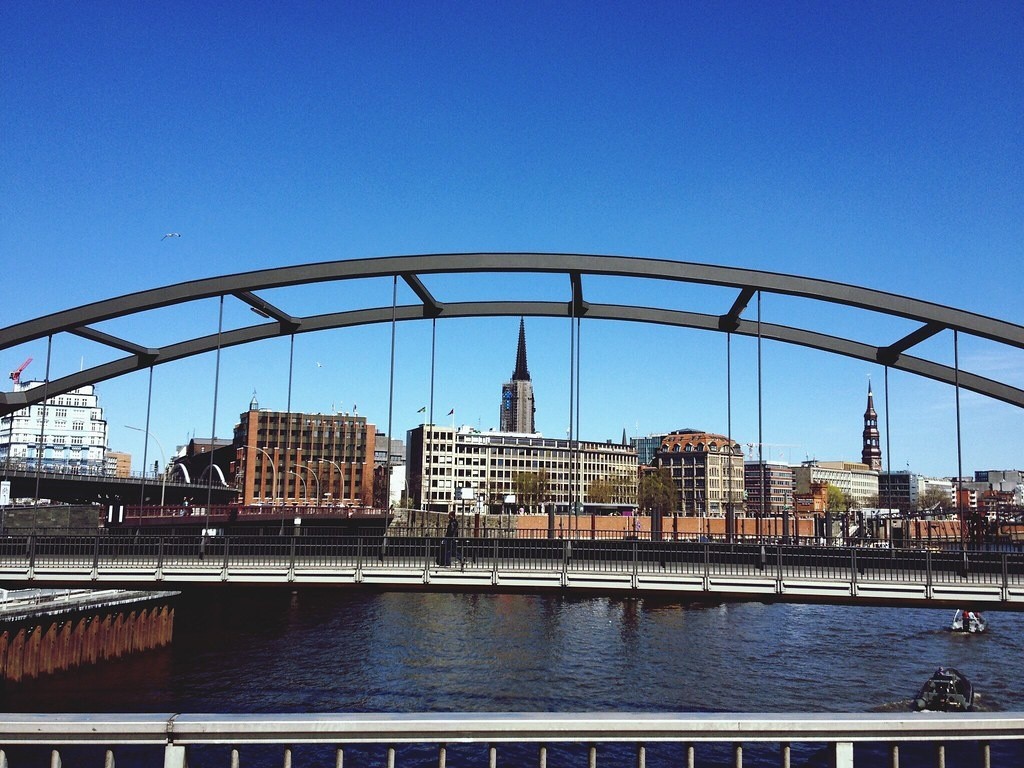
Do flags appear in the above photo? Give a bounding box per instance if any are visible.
[447,409,453,415]
[417,407,425,413]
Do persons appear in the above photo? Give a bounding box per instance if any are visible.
[923,666,948,712]
[443,511,466,567]
[961,610,984,632]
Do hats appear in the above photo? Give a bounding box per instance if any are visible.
[449,511,455,517]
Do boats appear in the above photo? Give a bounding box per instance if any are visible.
[950,608,986,632]
[914,666,975,714]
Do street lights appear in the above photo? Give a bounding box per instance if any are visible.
[243,445,276,506]
[318,458,344,506]
[124,425,167,517]
[287,470,307,506]
[294,463,319,507]
